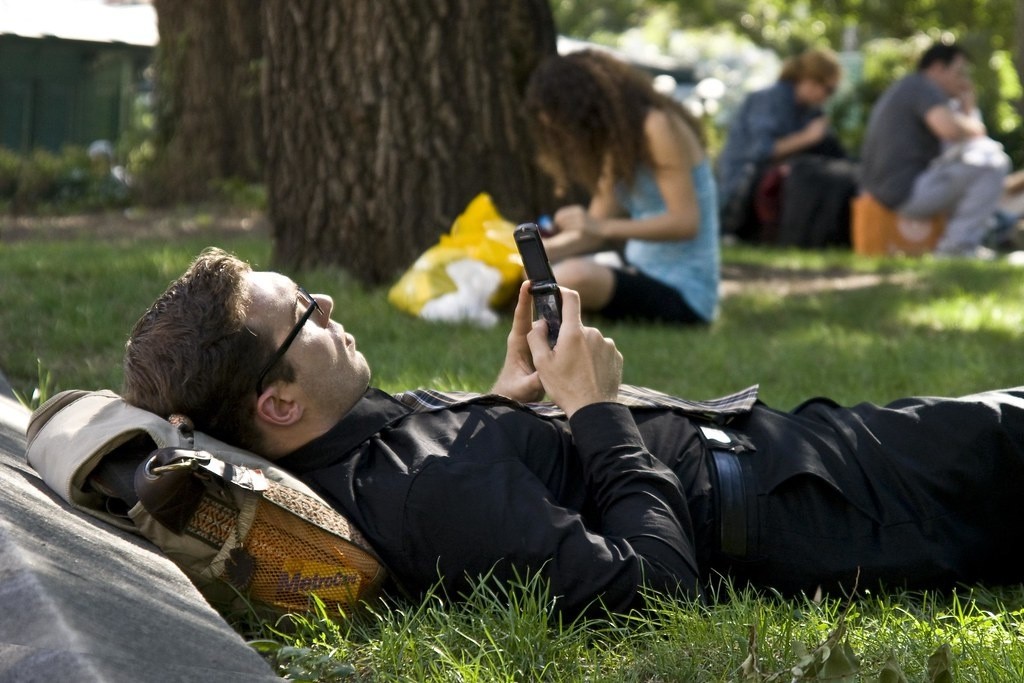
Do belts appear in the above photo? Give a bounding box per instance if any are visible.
[692,416,746,592]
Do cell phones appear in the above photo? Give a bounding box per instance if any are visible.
[512,223,563,352]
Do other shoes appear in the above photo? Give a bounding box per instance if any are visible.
[933,243,994,264]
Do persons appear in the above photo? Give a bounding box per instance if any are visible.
[524,49,720,328]
[125,250,1024,638]
[715,47,854,249]
[861,42,1011,260]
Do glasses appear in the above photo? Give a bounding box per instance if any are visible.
[255,280,325,398]
[817,77,836,97]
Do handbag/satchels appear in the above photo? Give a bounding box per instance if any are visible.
[26,390,386,634]
[388,193,524,329]
